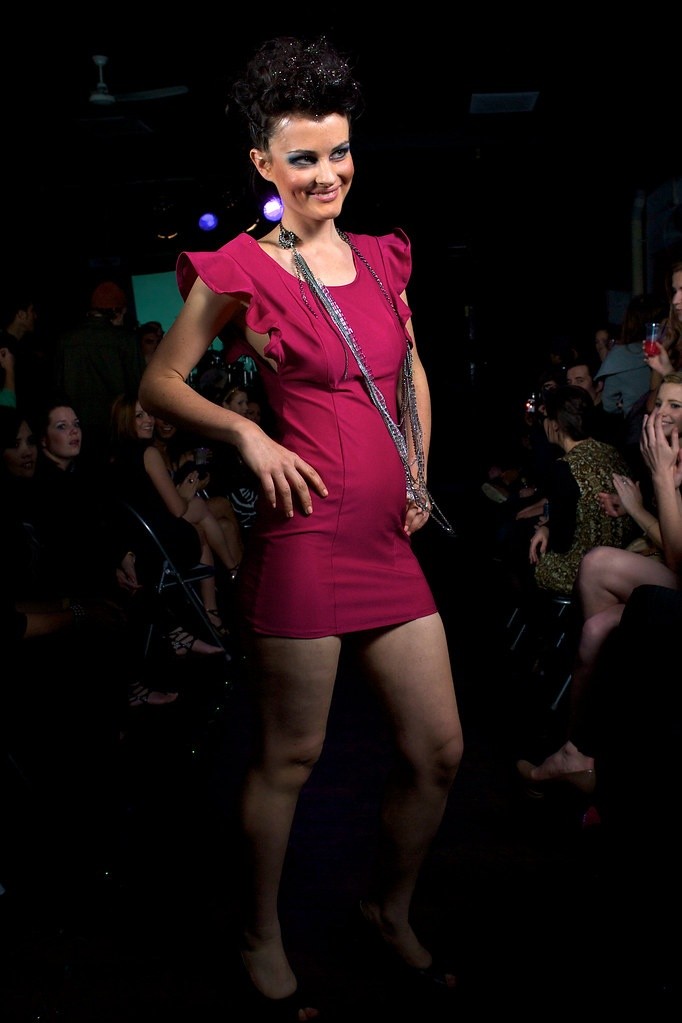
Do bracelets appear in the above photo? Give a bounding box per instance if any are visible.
[643,519,661,536]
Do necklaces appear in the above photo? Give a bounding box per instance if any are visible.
[277,221,456,536]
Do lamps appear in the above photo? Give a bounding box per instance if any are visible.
[89,55,116,105]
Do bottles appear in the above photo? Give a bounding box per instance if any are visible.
[543,503,549,516]
[521,477,530,489]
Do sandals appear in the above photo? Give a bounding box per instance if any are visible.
[165,628,226,668]
[126,679,185,712]
[226,564,241,586]
[204,608,230,639]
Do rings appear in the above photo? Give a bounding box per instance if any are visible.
[623,481,627,484]
[189,478,193,484]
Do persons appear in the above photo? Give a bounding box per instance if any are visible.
[137,37,465,1000]
[480,262,682,795]
[0,281,262,709]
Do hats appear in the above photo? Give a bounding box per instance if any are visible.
[92,280,125,310]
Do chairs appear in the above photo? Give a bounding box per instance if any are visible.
[506,575,583,713]
[114,500,237,669]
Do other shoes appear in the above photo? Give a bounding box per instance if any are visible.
[235,918,325,1023]
[352,887,459,991]
[517,757,597,794]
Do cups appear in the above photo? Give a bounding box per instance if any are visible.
[194,447,210,467]
[644,322,664,357]
